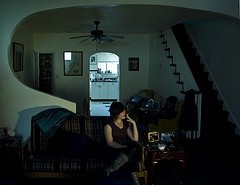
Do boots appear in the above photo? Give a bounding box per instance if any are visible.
[128,172,141,185]
[104,152,128,178]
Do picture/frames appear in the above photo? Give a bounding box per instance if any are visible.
[12,42,25,72]
[63,51,83,76]
[128,57,140,71]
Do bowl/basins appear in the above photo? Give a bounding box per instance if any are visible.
[158,144,167,150]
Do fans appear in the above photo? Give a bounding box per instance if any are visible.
[69,20,126,45]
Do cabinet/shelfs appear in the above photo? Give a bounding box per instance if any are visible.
[90,81,118,101]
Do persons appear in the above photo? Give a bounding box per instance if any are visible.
[102,102,140,185]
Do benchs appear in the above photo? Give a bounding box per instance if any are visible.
[17,116,148,185]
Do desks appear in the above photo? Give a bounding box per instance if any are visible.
[150,150,185,185]
